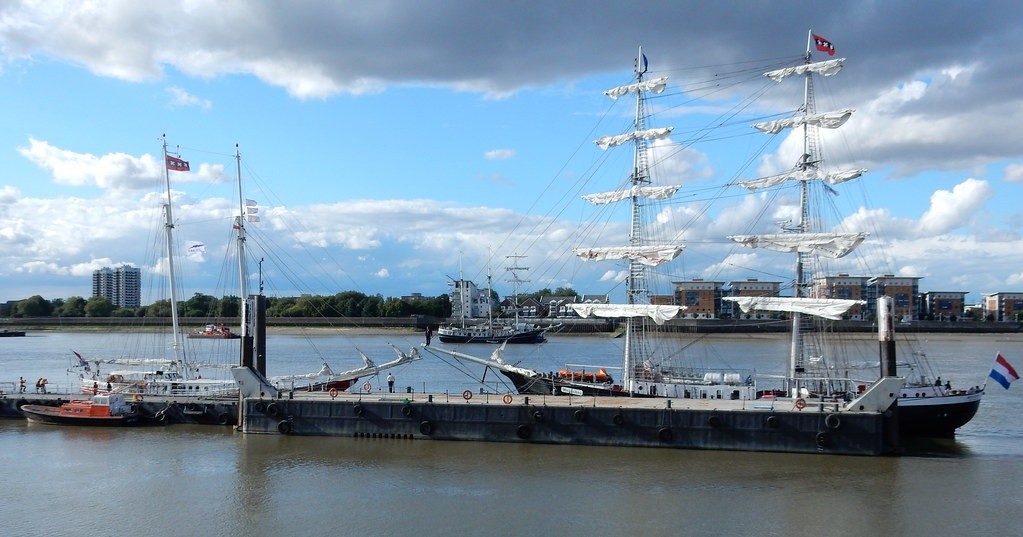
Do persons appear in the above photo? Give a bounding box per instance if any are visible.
[387,373,395,393]
[94,382,98,395]
[935,377,942,386]
[107,382,111,392]
[425,326,433,346]
[945,381,951,390]
[20,377,26,392]
[36,378,48,393]
[969,386,980,394]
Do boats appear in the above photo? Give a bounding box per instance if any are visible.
[559,366,611,382]
[186,322,242,339]
[19,392,142,427]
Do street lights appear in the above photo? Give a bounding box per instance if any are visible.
[917,294,922,320]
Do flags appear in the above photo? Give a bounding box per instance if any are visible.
[812,33,836,55]
[248,215,260,222]
[167,155,190,171]
[247,207,258,214]
[246,199,258,206]
[989,354,1020,390]
[233,225,240,229]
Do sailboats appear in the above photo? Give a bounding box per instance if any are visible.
[0,129,424,428]
[228,27,1023,456]
[435,243,564,345]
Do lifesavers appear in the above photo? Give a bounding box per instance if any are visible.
[363,383,372,391]
[188,403,196,411]
[767,416,777,426]
[135,394,144,401]
[255,402,265,412]
[533,411,544,424]
[278,420,291,434]
[329,388,338,397]
[503,395,513,404]
[516,424,531,439]
[217,414,228,425]
[574,410,582,420]
[708,416,721,427]
[613,414,624,426]
[462,390,472,400]
[267,403,281,418]
[353,405,363,415]
[401,405,411,416]
[816,413,842,448]
[657,428,674,442]
[420,421,432,435]
[795,398,806,409]
